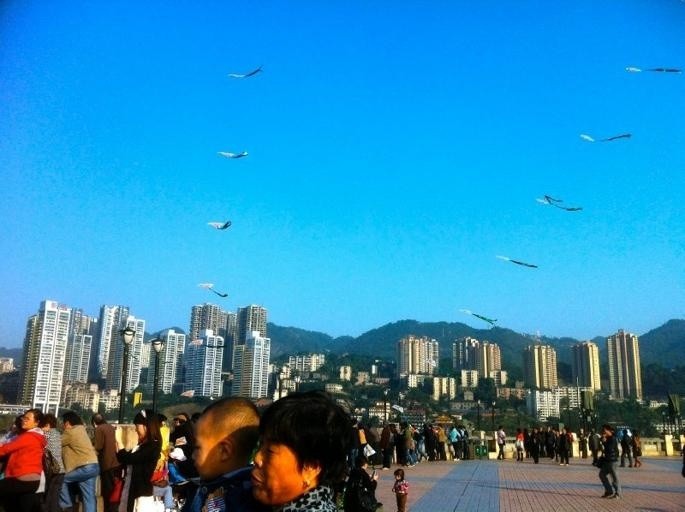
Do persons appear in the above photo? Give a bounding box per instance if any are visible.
[681,444,685,477]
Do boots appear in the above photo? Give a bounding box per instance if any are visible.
[517,453,523,462]
[497,451,506,460]
[634,459,642,468]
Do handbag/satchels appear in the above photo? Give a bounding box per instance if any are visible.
[42,447,60,477]
[592,452,605,468]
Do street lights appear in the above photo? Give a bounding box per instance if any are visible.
[383,387,388,422]
[491,399,496,430]
[151,332,165,413]
[578,407,599,436]
[396,389,404,422]
[228,370,234,396]
[660,411,684,435]
[119,322,135,424]
[477,398,482,430]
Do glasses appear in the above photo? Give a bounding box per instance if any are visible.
[174,418,186,424]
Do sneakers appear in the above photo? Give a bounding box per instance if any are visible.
[408,465,416,468]
[382,467,390,470]
[601,492,613,497]
[609,494,623,500]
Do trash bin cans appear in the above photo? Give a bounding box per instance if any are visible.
[473,438,489,460]
